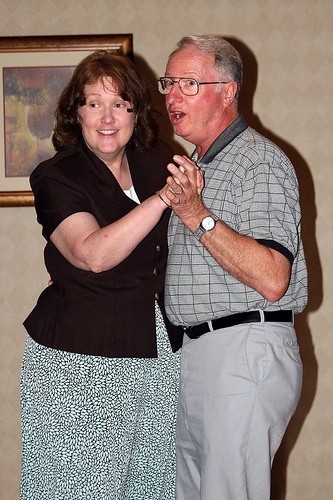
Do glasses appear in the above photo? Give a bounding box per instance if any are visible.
[157,76,230,96]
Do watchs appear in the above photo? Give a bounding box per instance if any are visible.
[193,213,219,241]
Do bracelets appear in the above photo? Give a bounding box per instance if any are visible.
[156,191,171,208]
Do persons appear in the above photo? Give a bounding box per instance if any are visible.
[19,49,204,500]
[46,34,310,500]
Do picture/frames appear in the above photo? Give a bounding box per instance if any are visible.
[0,32,134,207]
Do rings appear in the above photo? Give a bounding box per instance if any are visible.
[175,189,183,195]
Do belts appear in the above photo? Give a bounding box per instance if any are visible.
[183,309,294,339]
